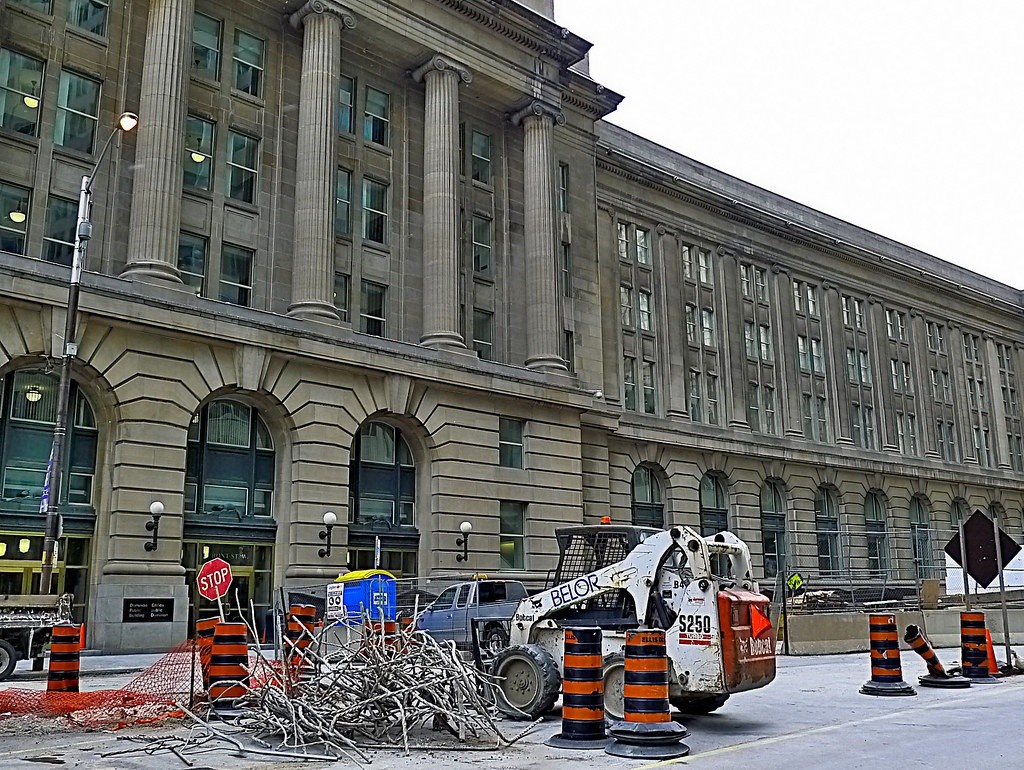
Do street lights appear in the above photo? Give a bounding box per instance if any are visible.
[31,112,138,671]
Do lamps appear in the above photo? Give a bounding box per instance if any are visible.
[25,385,43,402]
[9,199,26,224]
[191,137,206,162]
[0,537,31,558]
[317,510,338,558]
[23,81,40,110]
[144,501,164,552]
[456,520,472,562]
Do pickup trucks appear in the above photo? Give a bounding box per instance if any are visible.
[417,580,544,660]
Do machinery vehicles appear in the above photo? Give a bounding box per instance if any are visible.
[469,516,777,720]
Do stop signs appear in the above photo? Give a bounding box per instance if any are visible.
[196,558,233,601]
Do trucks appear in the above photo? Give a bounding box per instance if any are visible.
[0,594,59,683]
